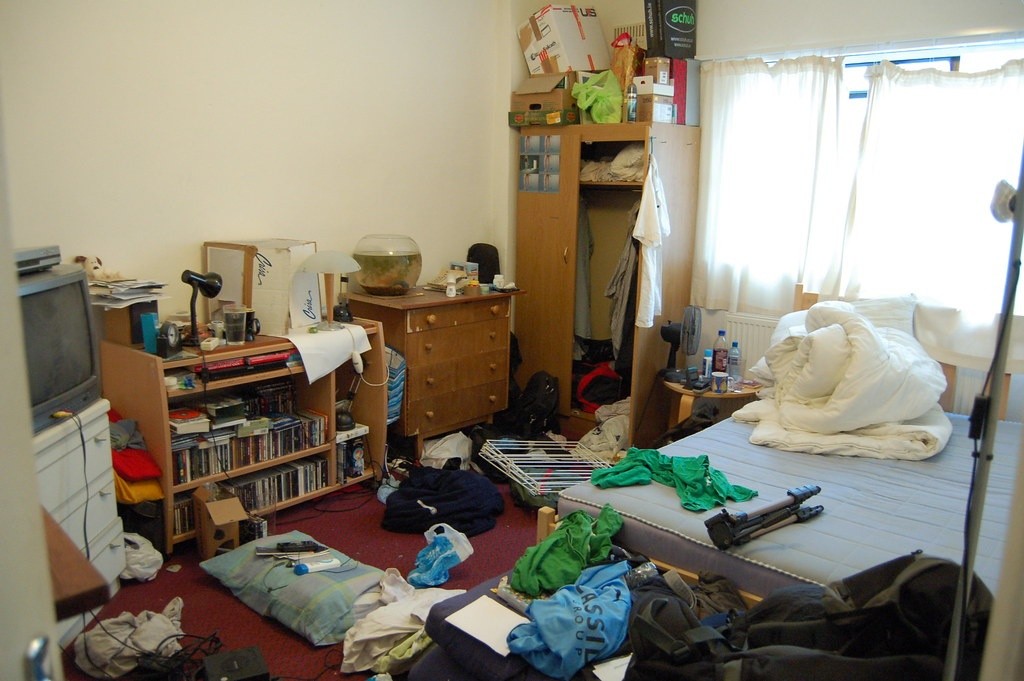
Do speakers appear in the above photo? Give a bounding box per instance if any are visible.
[92,297,158,349]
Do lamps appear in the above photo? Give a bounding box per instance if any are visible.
[180,268,225,351]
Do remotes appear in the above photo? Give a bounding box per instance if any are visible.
[200,337,220,351]
[277,541,319,552]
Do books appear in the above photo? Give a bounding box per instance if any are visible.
[173,490,195,535]
[218,454,329,513]
[88,285,165,294]
[427,268,470,290]
[185,349,303,372]
[168,385,329,485]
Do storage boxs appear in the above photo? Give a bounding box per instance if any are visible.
[193,483,249,557]
[101,291,162,349]
[507,0,702,128]
[449,260,478,279]
[200,238,322,336]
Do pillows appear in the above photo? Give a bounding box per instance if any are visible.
[198,529,399,648]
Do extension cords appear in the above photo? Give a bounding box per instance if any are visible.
[386,462,410,477]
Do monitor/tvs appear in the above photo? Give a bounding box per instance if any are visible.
[17,262,103,433]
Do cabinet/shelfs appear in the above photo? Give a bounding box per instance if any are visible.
[342,279,520,464]
[513,121,704,454]
[36,398,133,654]
[100,320,393,559]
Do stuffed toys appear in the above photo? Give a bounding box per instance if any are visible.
[75,255,121,280]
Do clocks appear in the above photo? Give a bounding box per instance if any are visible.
[156,320,177,361]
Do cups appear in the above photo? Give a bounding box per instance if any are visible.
[712,372,735,394]
[245,309,261,342]
[223,304,247,346]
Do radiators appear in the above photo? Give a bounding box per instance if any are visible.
[713,310,791,415]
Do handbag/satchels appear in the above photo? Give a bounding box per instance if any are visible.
[407,523,474,589]
[571,69,623,123]
[501,371,560,439]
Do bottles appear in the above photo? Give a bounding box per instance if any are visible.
[702,349,713,379]
[726,341,741,392]
[712,330,728,373]
[493,275,504,288]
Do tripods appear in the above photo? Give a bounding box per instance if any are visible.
[705,485,824,550]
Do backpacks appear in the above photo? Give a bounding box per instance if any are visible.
[621,549,993,681]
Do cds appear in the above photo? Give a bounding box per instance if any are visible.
[168,409,199,418]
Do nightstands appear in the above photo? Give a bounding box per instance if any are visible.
[660,375,761,433]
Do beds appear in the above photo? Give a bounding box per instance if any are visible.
[533,283,1024,625]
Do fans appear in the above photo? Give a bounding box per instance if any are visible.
[660,304,707,384]
